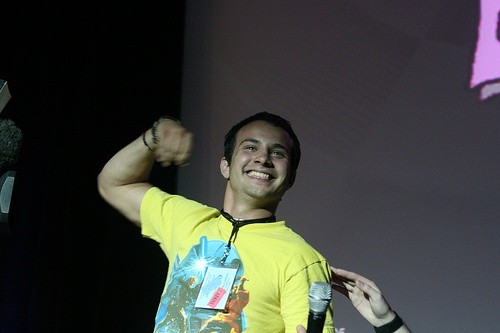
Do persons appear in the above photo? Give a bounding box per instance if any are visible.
[296,264,414,333]
[96,111,336,333]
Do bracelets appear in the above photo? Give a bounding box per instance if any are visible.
[142,128,153,152]
[150,117,182,144]
[374,310,405,333]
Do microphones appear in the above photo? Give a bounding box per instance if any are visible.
[306,281,332,333]
